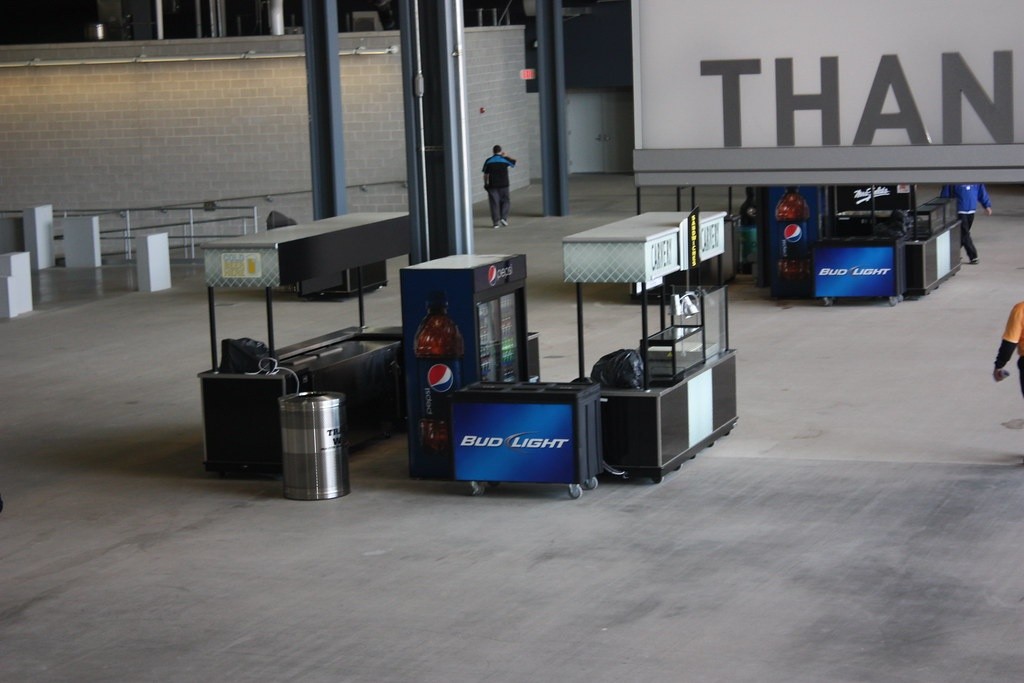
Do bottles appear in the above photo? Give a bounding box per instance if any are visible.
[477,293,519,383]
[776,185,813,294]
[414,287,464,458]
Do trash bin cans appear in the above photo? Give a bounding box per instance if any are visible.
[277,387,351,502]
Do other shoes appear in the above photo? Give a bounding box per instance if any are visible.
[494,225,499,228]
[501,218,508,225]
[970,258,979,264]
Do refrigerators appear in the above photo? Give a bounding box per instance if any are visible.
[759,184,839,301]
[400,253,529,483]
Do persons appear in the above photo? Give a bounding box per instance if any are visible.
[482,145,517,229]
[993,301,1024,466]
[940,182,992,264]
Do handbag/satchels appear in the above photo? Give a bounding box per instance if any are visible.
[591,349,643,388]
[219,338,268,373]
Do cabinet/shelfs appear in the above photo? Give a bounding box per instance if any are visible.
[195,207,410,481]
[562,210,737,478]
[830,185,961,295]
